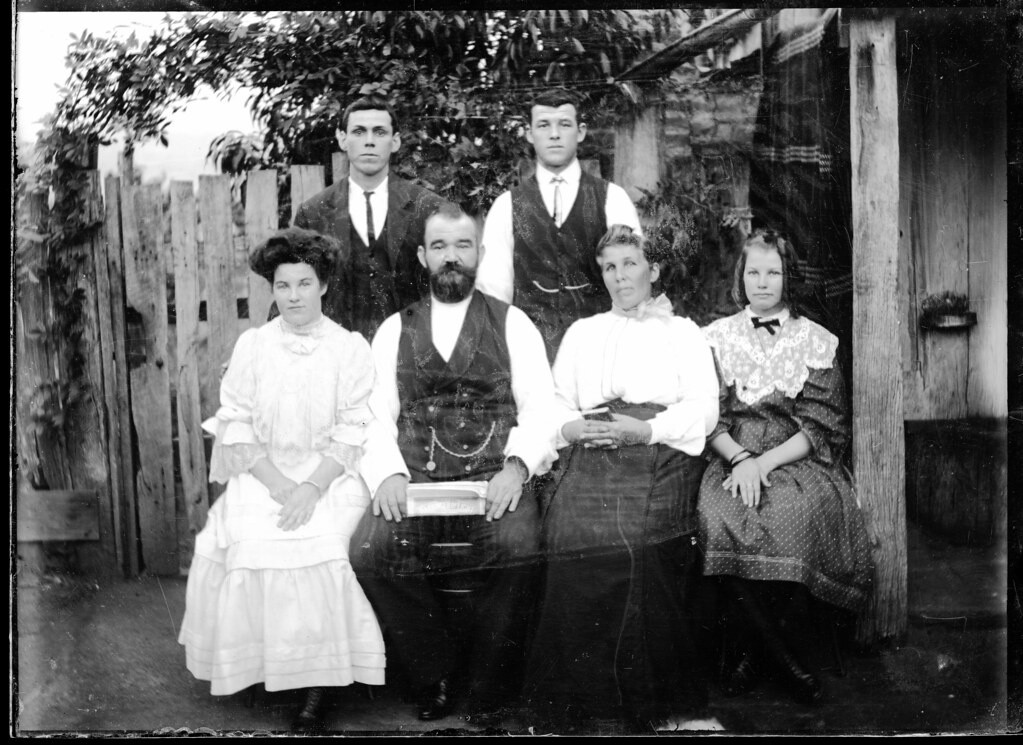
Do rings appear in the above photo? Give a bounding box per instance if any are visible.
[747,483,752,487]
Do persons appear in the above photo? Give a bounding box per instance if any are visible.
[177,224,388,732]
[474,89,645,367]
[551,223,720,734]
[357,195,557,728]
[699,229,874,705]
[267,97,449,345]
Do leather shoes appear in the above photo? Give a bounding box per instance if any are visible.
[418,679,456,720]
[781,652,822,702]
[725,653,760,696]
[291,688,327,732]
[465,688,496,726]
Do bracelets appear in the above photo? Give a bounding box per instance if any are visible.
[729,448,752,468]
[301,480,324,498]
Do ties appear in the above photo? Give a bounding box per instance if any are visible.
[364,191,376,247]
[553,176,565,228]
